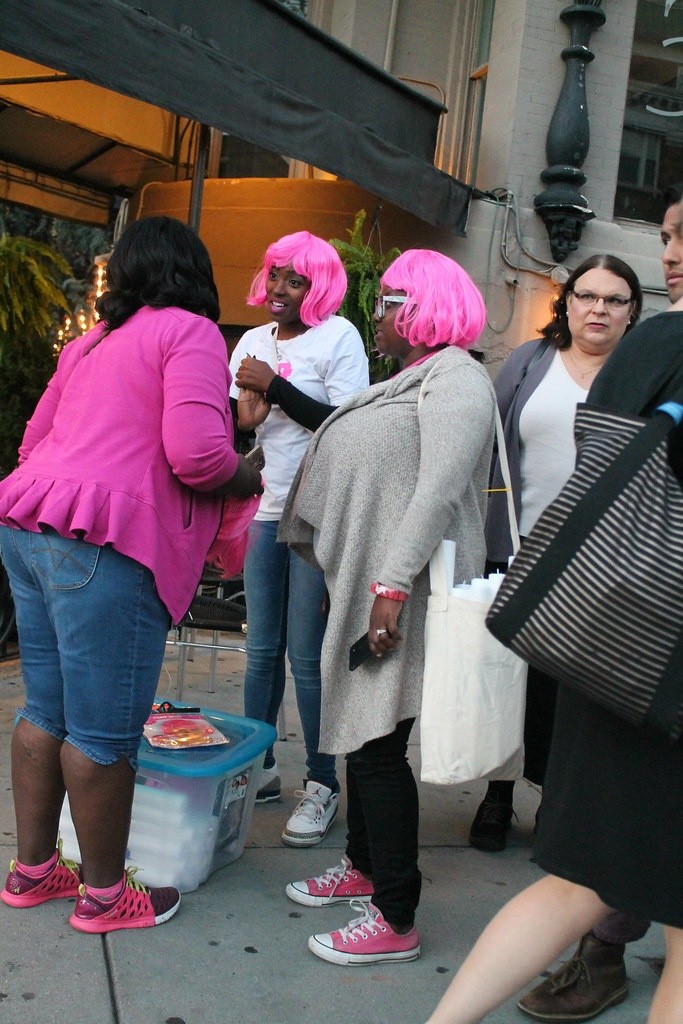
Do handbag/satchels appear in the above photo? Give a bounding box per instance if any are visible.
[486,383,683,717]
[420,575,527,784]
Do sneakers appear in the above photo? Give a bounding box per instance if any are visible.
[308,898,421,967]
[285,853,376,907]
[283,778,340,847]
[255,758,282,803]
[0,830,85,908]
[69,867,181,934]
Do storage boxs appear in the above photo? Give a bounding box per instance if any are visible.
[12,697,278,894]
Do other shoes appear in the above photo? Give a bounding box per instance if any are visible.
[468,791,519,852]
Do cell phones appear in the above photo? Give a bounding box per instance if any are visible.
[349,629,392,671]
[243,445,266,473]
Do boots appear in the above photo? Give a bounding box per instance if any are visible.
[518,931,630,1024]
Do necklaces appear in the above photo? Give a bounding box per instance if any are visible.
[272,324,306,362]
[567,349,607,379]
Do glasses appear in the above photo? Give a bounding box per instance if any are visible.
[566,289,633,309]
[375,295,419,318]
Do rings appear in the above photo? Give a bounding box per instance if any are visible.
[377,629,387,638]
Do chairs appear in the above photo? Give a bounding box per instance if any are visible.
[187,563,245,662]
[165,595,248,703]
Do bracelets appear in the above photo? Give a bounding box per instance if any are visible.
[370,585,409,602]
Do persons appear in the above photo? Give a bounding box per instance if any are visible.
[221,230,369,850]
[284,247,497,970]
[424,294,683,1024]
[514,189,683,1023]
[0,214,264,935]
[468,252,644,852]
